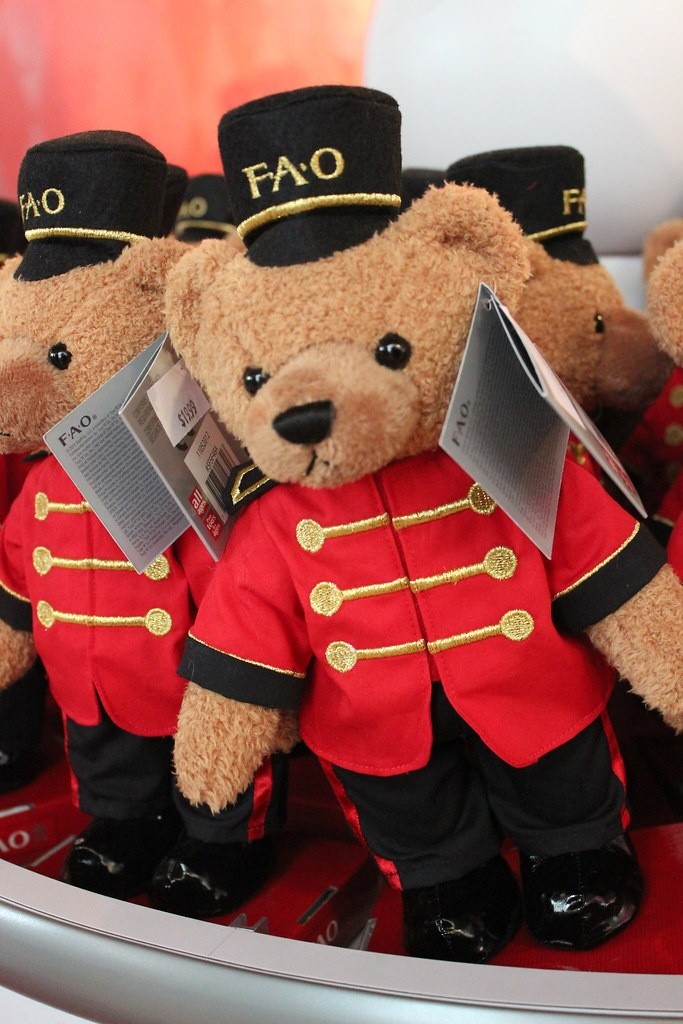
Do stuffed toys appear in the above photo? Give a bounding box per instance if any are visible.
[0,129,296,919]
[0,86,683,964]
